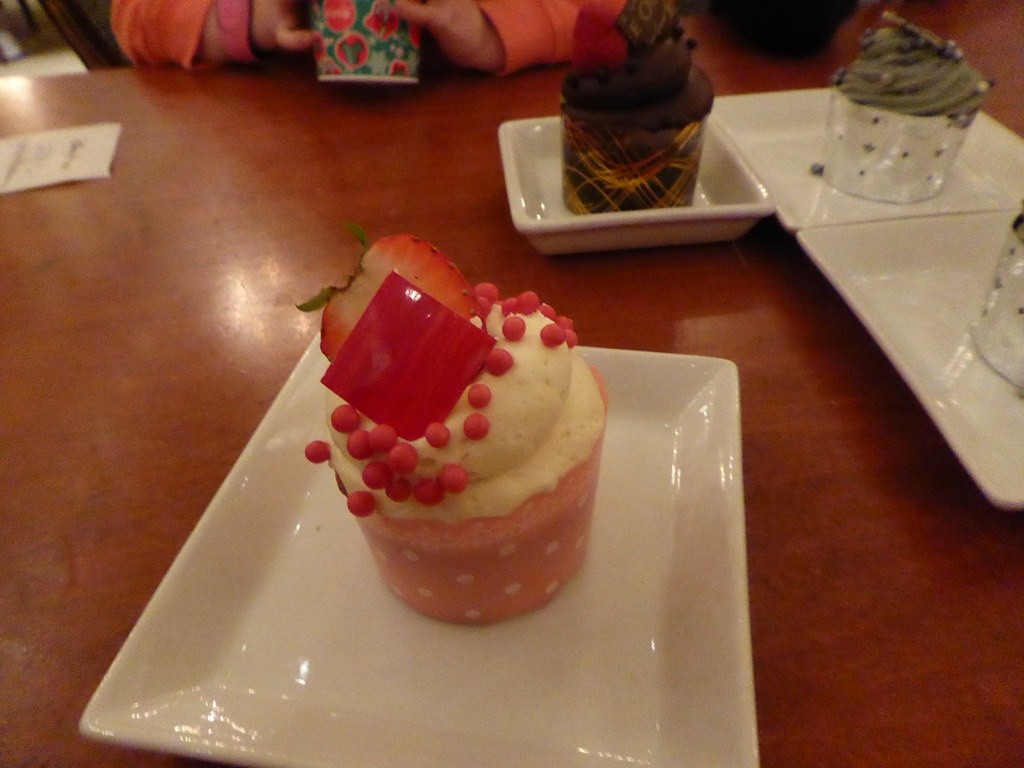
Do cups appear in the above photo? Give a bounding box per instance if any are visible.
[307,0,422,84]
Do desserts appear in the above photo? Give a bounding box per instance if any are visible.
[823,10,997,204]
[294,224,610,628]
[974,198,1023,388]
[558,0,714,216]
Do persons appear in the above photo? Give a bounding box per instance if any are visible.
[111,0,630,78]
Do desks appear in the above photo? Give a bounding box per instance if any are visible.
[0,0,1024,768]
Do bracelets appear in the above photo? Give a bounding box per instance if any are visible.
[218,0,257,63]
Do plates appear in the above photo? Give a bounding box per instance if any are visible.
[709,85,1024,232]
[795,208,1024,511]
[498,114,777,256]
[77,332,760,768]
